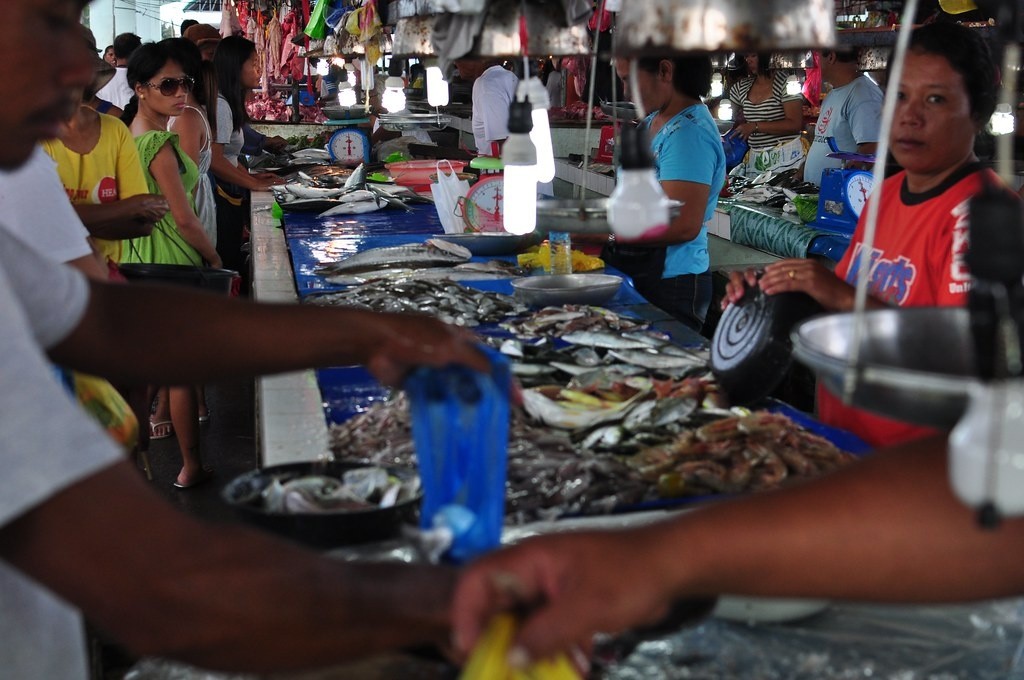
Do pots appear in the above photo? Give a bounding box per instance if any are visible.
[710,270,803,409]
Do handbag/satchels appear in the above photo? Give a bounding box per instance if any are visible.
[430,159,471,235]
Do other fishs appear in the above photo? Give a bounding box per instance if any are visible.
[288,156,326,164]
[486,323,711,426]
[325,258,530,285]
[599,95,636,109]
[291,147,330,159]
[269,162,433,219]
[312,237,473,276]
[303,276,521,328]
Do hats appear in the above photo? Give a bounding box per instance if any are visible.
[81,24,116,93]
[183,23,222,48]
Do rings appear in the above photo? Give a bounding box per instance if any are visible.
[788,270,796,278]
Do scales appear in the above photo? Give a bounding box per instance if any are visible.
[461,158,503,234]
[321,107,370,165]
[285,80,314,105]
[805,136,875,233]
[594,100,638,164]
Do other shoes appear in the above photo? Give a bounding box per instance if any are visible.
[170,469,197,503]
[198,409,210,428]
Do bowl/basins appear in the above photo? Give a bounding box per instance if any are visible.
[118,263,238,296]
[511,274,624,309]
[429,173,478,188]
[384,159,468,192]
[432,231,545,254]
[217,460,424,540]
[600,101,637,120]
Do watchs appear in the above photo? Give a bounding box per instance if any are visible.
[607,233,617,247]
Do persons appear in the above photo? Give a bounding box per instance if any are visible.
[722,50,802,149]
[721,22,1024,451]
[542,61,562,110]
[447,435,1023,679]
[453,55,555,196]
[792,44,884,189]
[0,0,525,680]
[569,50,726,335]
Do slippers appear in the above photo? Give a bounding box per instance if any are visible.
[149,420,172,440]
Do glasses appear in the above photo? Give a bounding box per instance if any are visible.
[142,76,195,97]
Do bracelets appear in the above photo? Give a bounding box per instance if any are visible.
[754,121,759,133]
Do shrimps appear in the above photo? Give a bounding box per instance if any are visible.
[629,410,845,500]
[328,392,639,525]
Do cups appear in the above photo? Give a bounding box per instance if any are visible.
[550,231,572,274]
